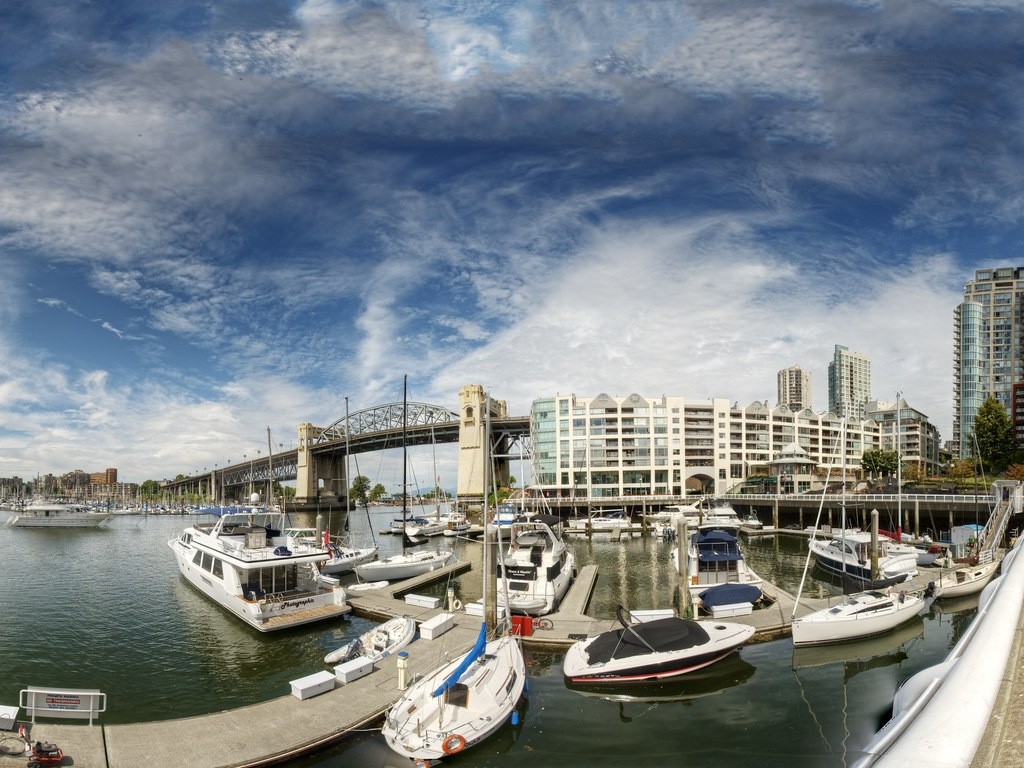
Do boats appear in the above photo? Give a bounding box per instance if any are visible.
[8,502,110,529]
[563,603,757,688]
[671,523,764,620]
[486,497,776,546]
[322,614,416,669]
[167,491,352,635]
[805,531,920,591]
[562,658,756,723]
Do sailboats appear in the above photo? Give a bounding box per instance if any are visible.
[925,433,1003,599]
[300,396,380,574]
[847,392,941,567]
[930,593,979,640]
[384,423,474,539]
[251,424,331,548]
[0,483,283,517]
[347,371,454,582]
[788,613,927,767]
[378,384,532,768]
[790,416,927,649]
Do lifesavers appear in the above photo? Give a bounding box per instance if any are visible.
[442,734,466,754]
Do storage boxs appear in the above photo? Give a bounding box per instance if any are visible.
[0,705,20,730]
[322,576,340,587]
[333,655,374,684]
[418,613,455,640]
[711,601,754,618]
[569,520,860,535]
[464,603,506,619]
[629,609,675,624]
[288,670,337,700]
[404,593,440,609]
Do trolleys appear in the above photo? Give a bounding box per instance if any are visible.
[19,724,64,768]
